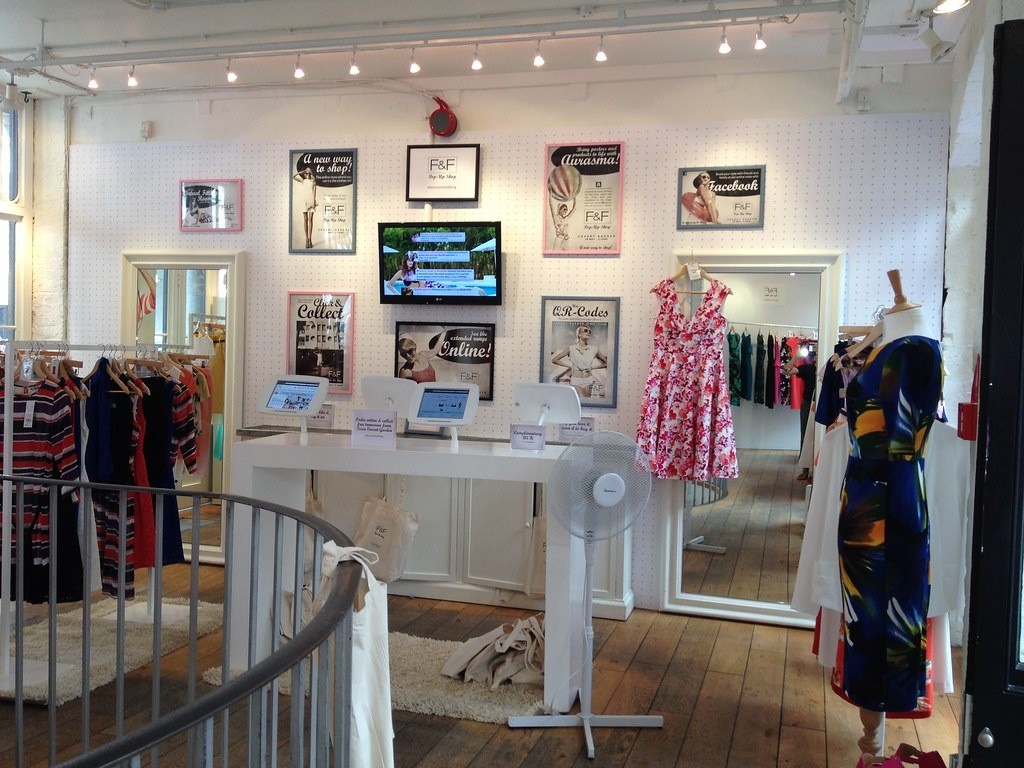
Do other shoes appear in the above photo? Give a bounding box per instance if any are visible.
[306,242,312,248]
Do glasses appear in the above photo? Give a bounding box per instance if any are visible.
[699,176,709,181]
[581,329,591,334]
[400,348,416,358]
[563,208,568,211]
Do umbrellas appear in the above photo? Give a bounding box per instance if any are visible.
[383,246,399,253]
[471,238,496,269]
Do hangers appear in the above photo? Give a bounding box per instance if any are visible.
[193,314,225,341]
[1,340,204,400]
[837,304,888,360]
[650,248,734,298]
[730,323,819,344]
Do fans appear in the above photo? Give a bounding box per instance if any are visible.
[507,428,664,756]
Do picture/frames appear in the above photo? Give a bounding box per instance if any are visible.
[286,141,768,408]
[180,179,244,232]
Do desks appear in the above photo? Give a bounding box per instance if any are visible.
[228,433,594,712]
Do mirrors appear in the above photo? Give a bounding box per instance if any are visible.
[659,250,845,630]
[118,249,248,563]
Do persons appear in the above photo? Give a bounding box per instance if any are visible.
[552,325,608,398]
[386,252,484,295]
[549,188,576,251]
[185,189,232,227]
[136,268,156,333]
[782,344,818,479]
[829,306,943,751]
[298,322,336,376]
[683,172,721,224]
[293,168,318,248]
[399,326,447,384]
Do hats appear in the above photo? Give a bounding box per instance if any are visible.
[557,203,567,214]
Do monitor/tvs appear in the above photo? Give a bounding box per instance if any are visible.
[362,376,418,416]
[378,222,502,306]
[513,383,581,424]
[408,382,480,426]
[257,374,329,417]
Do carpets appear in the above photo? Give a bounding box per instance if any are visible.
[0,591,224,707]
[203,624,552,725]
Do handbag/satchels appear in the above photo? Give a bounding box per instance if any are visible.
[440,613,546,687]
[353,478,420,583]
[310,540,394,768]
[303,475,324,573]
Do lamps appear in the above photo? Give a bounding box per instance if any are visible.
[5,71,18,99]
[88,20,769,90]
[914,0,970,64]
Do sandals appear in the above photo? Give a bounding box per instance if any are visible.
[856,744,946,768]
